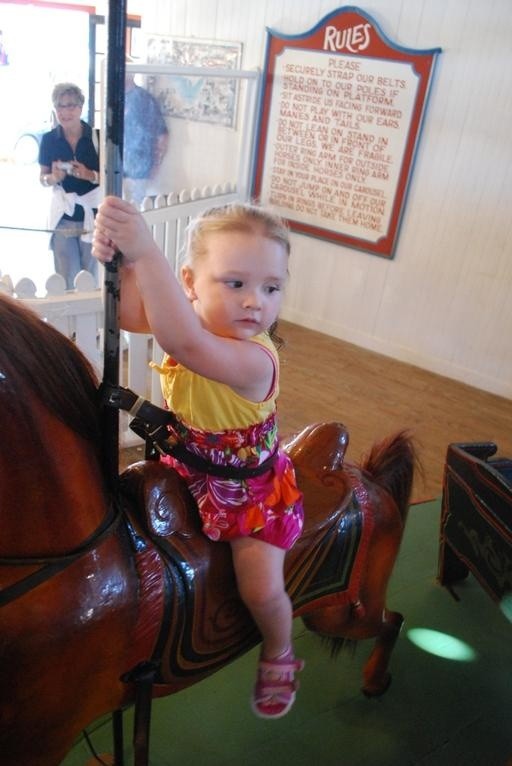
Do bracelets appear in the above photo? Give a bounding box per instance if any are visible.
[43,173,51,187]
[90,171,100,184]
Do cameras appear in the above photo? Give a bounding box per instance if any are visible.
[60,162,78,175]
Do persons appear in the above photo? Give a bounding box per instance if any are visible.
[90,193,307,721]
[121,70,167,208]
[38,83,100,290]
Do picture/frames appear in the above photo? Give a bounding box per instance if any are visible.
[143,36,243,131]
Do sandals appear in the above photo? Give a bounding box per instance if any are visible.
[251,642,304,720]
